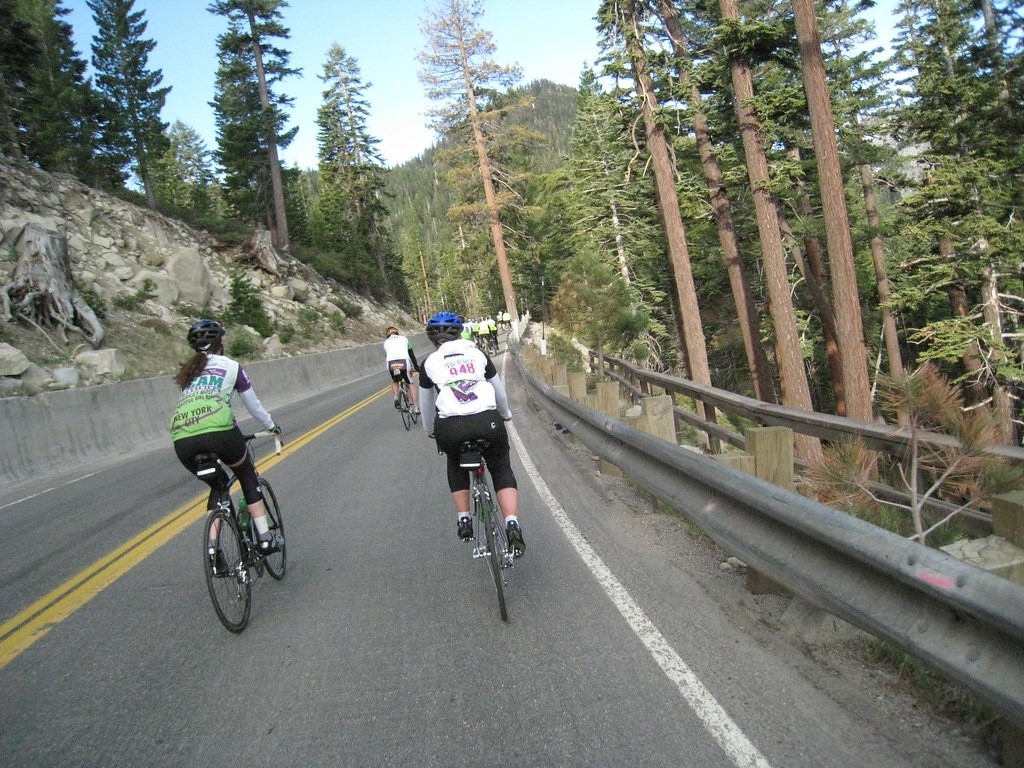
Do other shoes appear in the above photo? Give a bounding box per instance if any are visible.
[415,409,421,414]
[394,398,399,408]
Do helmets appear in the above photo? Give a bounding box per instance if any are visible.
[187,318,226,349]
[386,327,398,337]
[427,311,464,339]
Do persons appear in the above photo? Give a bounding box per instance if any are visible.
[168,318,284,574]
[382,326,422,415]
[461,316,499,354]
[417,311,526,557]
[496,311,512,330]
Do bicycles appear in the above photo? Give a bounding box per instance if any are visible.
[190,427,288,635]
[430,417,525,621]
[470,322,511,357]
[389,367,423,431]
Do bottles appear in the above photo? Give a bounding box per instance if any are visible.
[237,496,250,530]
[479,498,493,523]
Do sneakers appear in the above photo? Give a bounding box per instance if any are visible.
[258,532,284,553]
[505,520,524,556]
[457,517,474,539]
[208,547,227,571]
